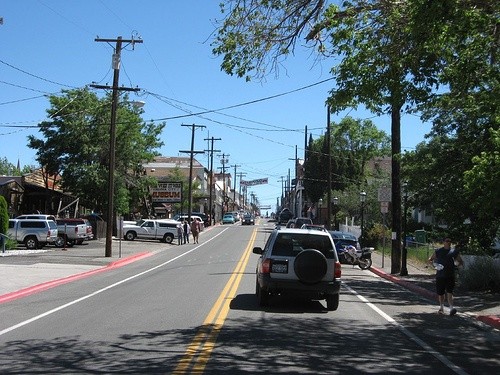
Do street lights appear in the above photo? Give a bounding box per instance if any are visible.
[359,189,366,240]
[333,196,339,230]
[318,197,323,223]
[105,100,146,257]
[205,197,207,212]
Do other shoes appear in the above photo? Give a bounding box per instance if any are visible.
[438,308,444,314]
[449,308,457,316]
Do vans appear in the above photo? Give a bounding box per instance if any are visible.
[15,214,87,247]
[294,217,313,228]
[177,215,204,231]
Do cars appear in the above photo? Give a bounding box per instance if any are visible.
[301,223,328,232]
[286,219,296,227]
[56,218,94,245]
[241,214,255,225]
[226,211,241,221]
[223,215,235,224]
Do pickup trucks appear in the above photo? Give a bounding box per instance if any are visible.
[6,218,59,248]
[123,219,190,242]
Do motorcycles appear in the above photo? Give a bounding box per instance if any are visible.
[336,240,375,270]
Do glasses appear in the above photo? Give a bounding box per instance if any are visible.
[444,240,452,242]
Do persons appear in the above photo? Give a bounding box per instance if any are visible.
[429,240,457,317]
[191,218,201,244]
[183,221,191,244]
[176,222,184,246]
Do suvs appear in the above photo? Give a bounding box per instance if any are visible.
[328,230,360,262]
[252,225,342,311]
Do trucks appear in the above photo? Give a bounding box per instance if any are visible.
[172,212,208,223]
[279,208,293,224]
